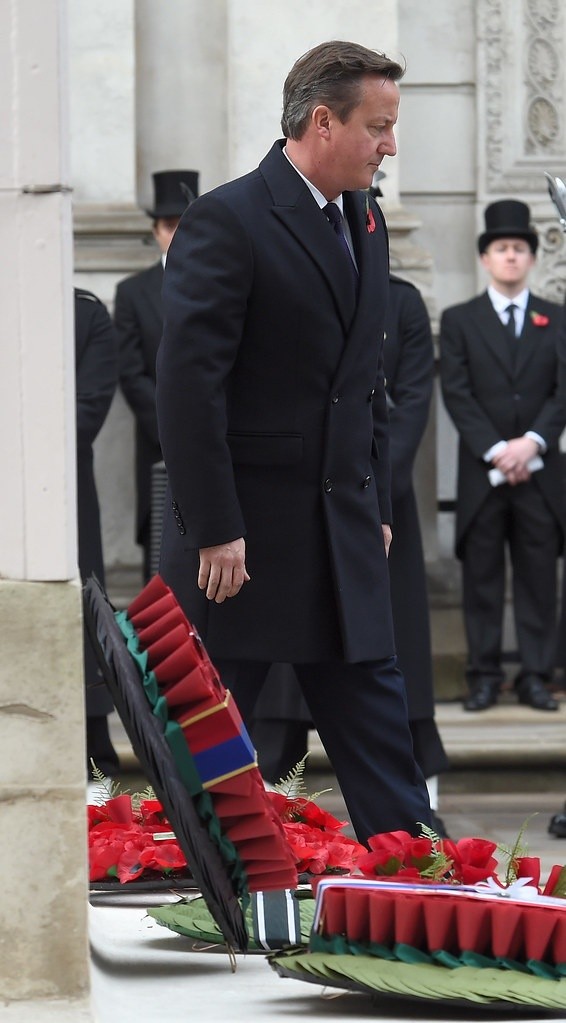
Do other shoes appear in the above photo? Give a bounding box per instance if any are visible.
[514,677,558,710]
[87,718,121,774]
[465,687,496,710]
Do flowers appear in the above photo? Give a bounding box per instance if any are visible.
[87,570,566,969]
[524,304,551,330]
[365,196,379,235]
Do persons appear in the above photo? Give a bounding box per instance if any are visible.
[440,200,566,836]
[75,169,458,813]
[156,40,453,855]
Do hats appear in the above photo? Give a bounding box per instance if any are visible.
[478,199,537,254]
[144,170,199,219]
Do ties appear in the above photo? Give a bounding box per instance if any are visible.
[322,202,359,307]
[505,303,515,337]
